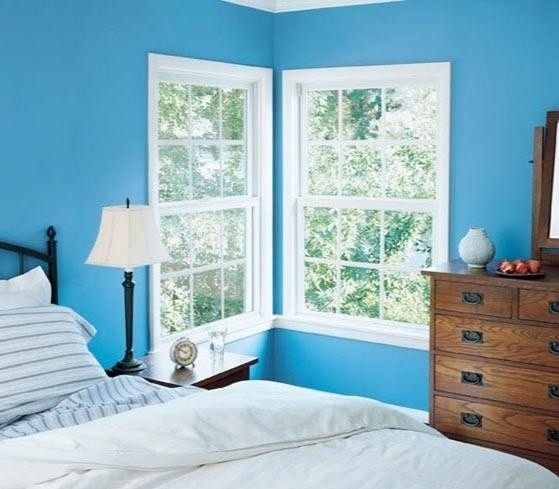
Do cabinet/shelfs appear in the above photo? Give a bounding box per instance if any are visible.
[422,259,559,476]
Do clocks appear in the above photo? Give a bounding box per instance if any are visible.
[170,337,198,370]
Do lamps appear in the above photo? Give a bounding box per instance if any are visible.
[84,197,174,373]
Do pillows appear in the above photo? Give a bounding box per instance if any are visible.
[0,264,112,430]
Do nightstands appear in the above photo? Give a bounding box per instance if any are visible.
[105,346,260,389]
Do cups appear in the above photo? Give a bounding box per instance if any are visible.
[208,326,227,357]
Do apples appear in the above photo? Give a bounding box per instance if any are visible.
[500,259,541,274]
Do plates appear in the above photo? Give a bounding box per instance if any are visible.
[495,268,547,278]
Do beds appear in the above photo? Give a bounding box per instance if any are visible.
[0,224,559,488]
